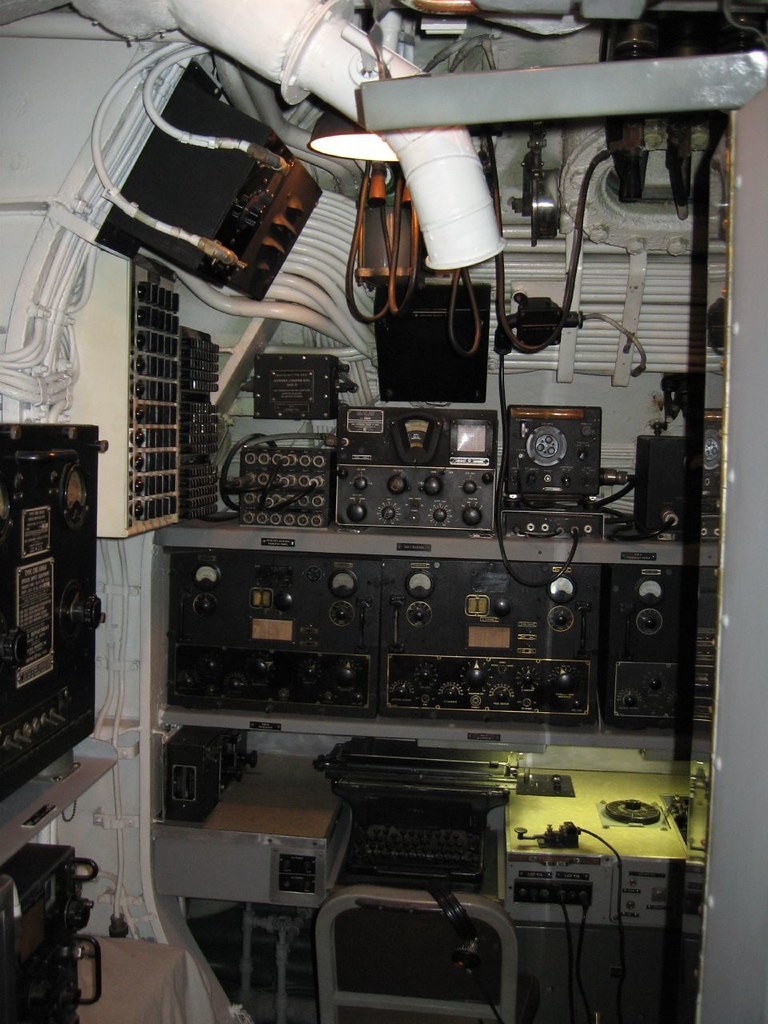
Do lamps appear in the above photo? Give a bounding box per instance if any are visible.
[309,107,399,162]
